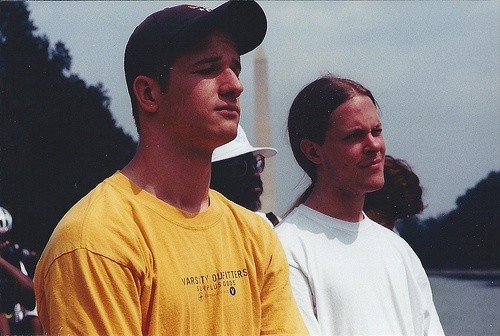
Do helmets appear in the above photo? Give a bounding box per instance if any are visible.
[0,207,13,233]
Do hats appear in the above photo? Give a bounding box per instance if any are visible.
[212,124,279,163]
[124,0,268,69]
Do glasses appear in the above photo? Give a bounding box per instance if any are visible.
[233,154,265,173]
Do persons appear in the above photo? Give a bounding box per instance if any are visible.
[0,206,41,336]
[211,152,282,226]
[32,32,308,336]
[273,76,445,336]
